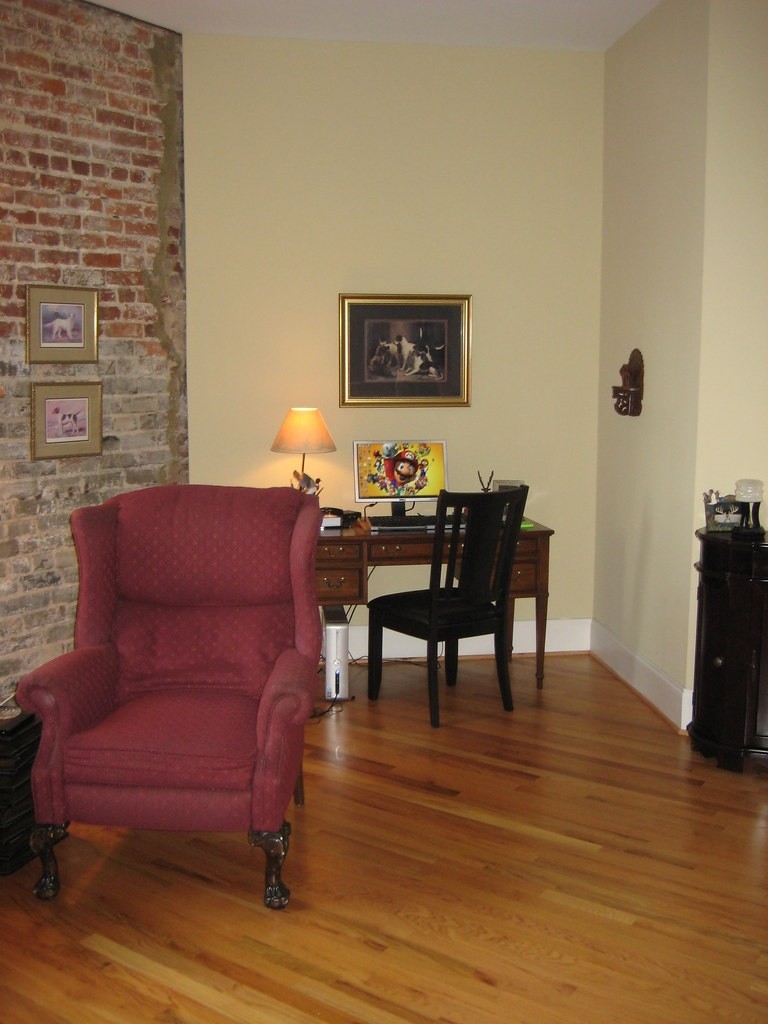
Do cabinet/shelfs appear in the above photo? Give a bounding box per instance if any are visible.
[686,528,768,772]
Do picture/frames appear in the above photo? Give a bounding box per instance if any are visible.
[339,292,473,409]
[24,284,99,362]
[30,382,104,457]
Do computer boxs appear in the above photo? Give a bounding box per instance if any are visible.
[321,605,350,700]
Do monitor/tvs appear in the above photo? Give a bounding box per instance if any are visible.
[353,440,449,516]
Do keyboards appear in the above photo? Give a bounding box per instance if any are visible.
[368,515,467,530]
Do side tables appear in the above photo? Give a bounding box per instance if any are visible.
[0,692,69,877]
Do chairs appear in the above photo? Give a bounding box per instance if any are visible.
[15,484,322,907]
[367,485,530,727]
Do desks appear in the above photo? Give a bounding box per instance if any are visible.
[315,515,554,704]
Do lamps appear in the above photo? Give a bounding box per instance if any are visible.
[269,405,338,494]
